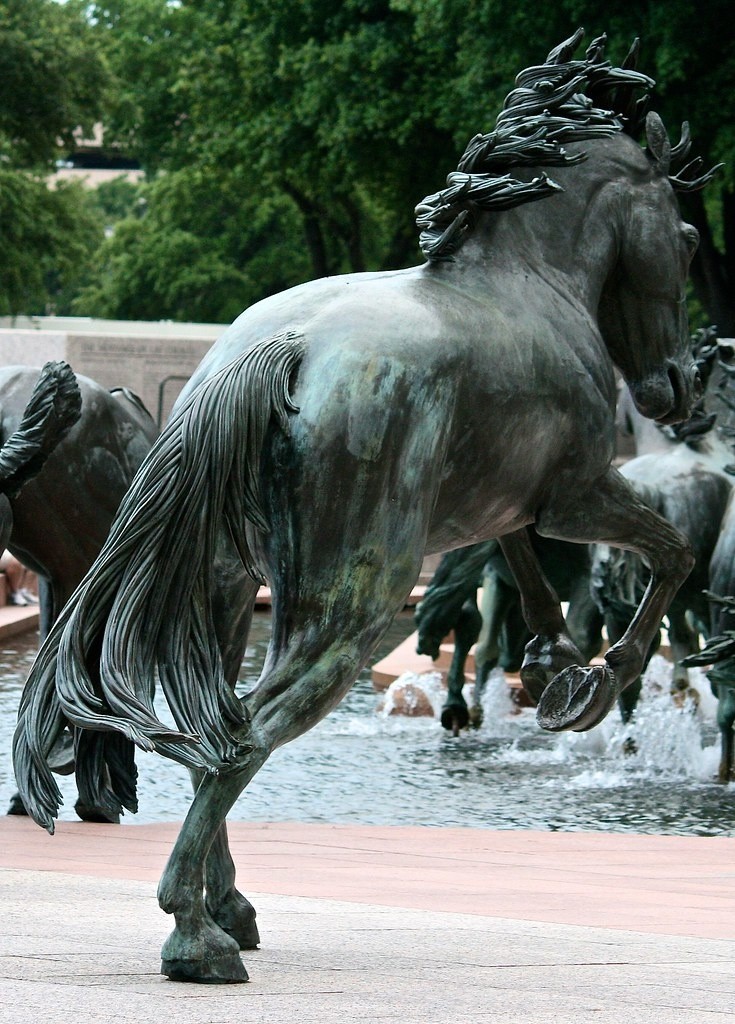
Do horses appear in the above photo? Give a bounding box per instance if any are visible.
[0,359,171,831]
[408,317,735,743]
[8,26,728,989]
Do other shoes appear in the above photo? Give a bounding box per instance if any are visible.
[9,591,28,606]
[22,590,39,604]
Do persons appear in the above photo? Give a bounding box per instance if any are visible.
[0,549,39,607]
